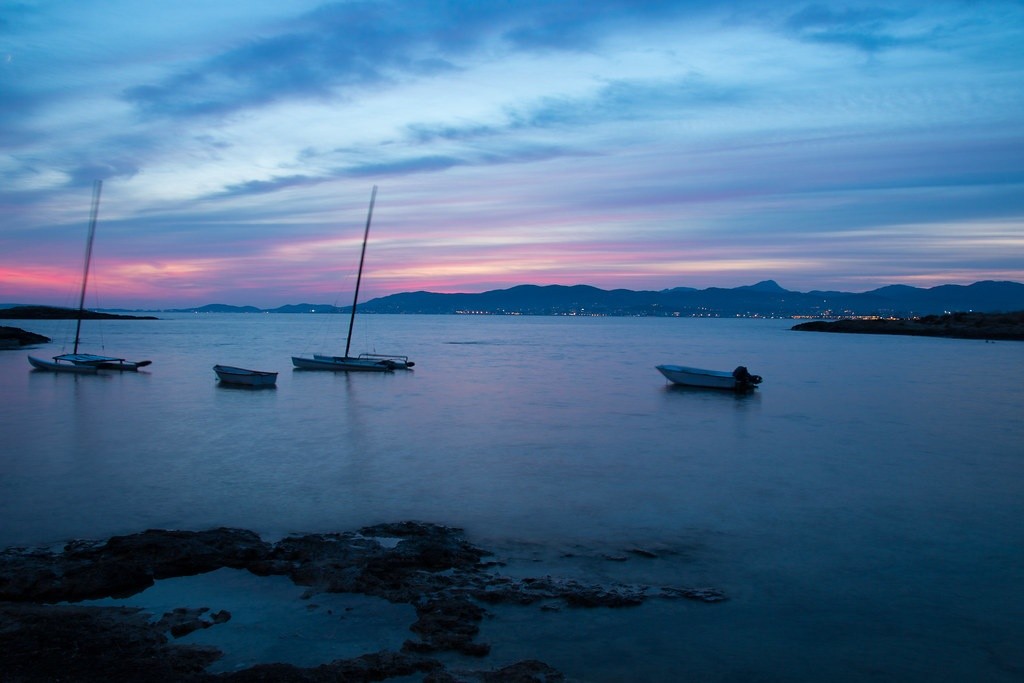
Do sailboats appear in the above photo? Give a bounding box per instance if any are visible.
[291,185,415,371]
[26,179,153,372]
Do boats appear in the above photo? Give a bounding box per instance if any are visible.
[213,364,280,387]
[654,364,763,395]
